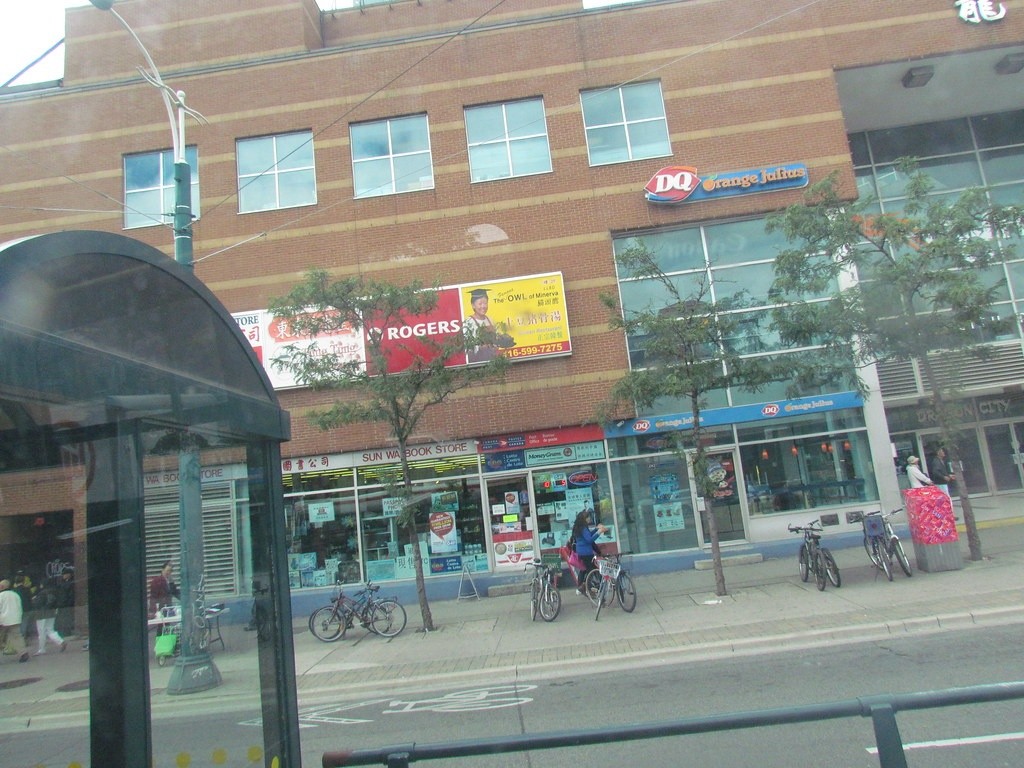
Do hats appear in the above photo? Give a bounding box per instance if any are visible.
[907,456,919,463]
[467,288,492,298]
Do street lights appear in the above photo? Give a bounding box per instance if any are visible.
[93,0,208,656]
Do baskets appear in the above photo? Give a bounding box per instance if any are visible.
[598,560,620,579]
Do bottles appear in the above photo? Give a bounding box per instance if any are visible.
[464,544,482,556]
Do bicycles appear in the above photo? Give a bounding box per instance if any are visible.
[585,554,621,621]
[849,511,894,582]
[788,523,828,591]
[878,508,912,578]
[805,519,842,587]
[308,579,393,641]
[311,577,406,642]
[523,557,563,621]
[599,551,638,613]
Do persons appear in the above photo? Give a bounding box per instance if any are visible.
[570,510,609,595]
[906,456,934,489]
[462,289,500,363]
[147,564,181,657]
[931,446,960,522]
[0,569,74,663]
[583,500,595,526]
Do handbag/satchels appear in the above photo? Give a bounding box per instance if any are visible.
[155,627,177,656]
[569,551,586,571]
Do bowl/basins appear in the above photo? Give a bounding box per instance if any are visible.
[569,471,598,486]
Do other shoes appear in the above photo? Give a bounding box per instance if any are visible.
[579,584,587,597]
[60,641,67,652]
[33,651,46,656]
[954,517,959,521]
[19,653,29,662]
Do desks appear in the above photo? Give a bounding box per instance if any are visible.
[147,608,229,651]
